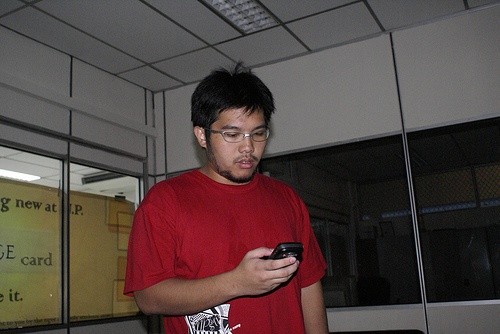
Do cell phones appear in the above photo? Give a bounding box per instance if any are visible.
[269,242,304,262]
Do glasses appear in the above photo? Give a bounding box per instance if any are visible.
[203,128,270,143]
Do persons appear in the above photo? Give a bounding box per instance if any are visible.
[123,61,329,334]
